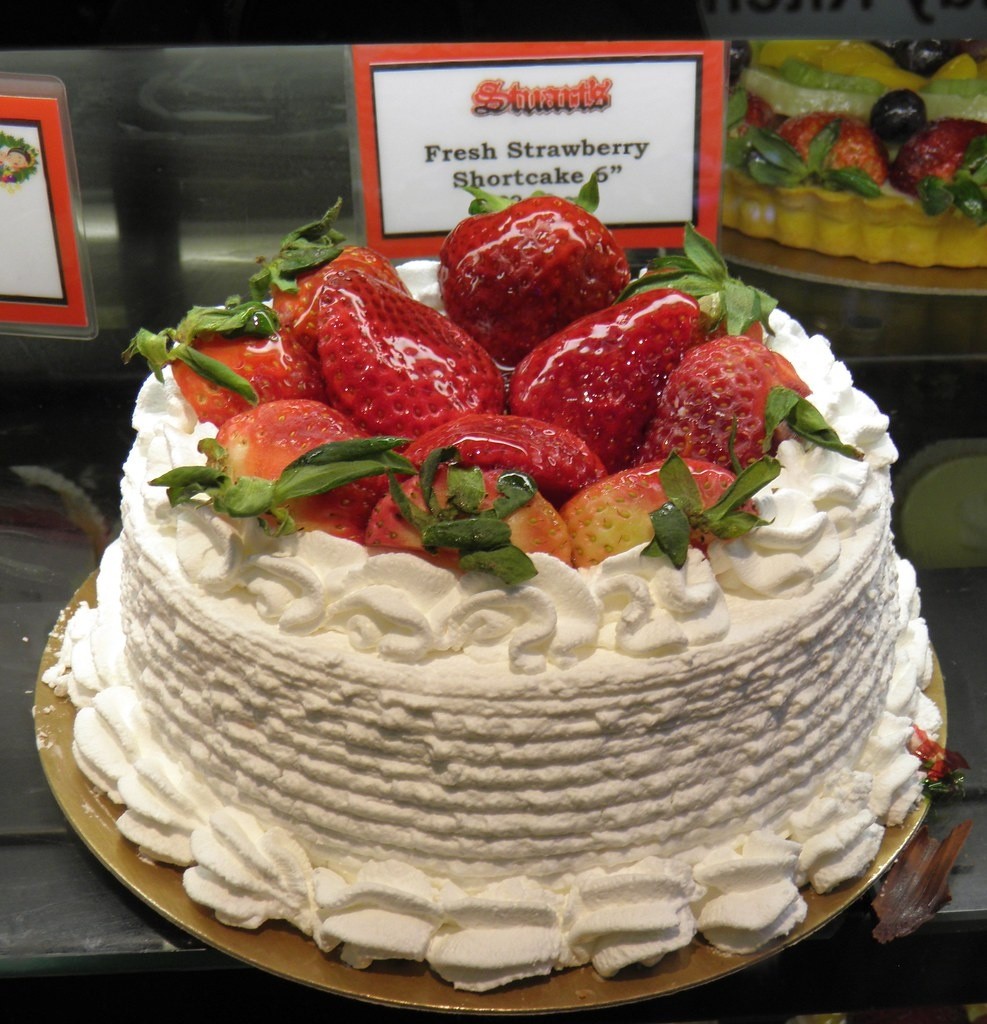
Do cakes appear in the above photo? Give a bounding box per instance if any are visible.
[36,164,947,989]
[714,31,987,270]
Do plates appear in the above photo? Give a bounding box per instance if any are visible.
[33,570,948,1016]
[722,226,987,296]
